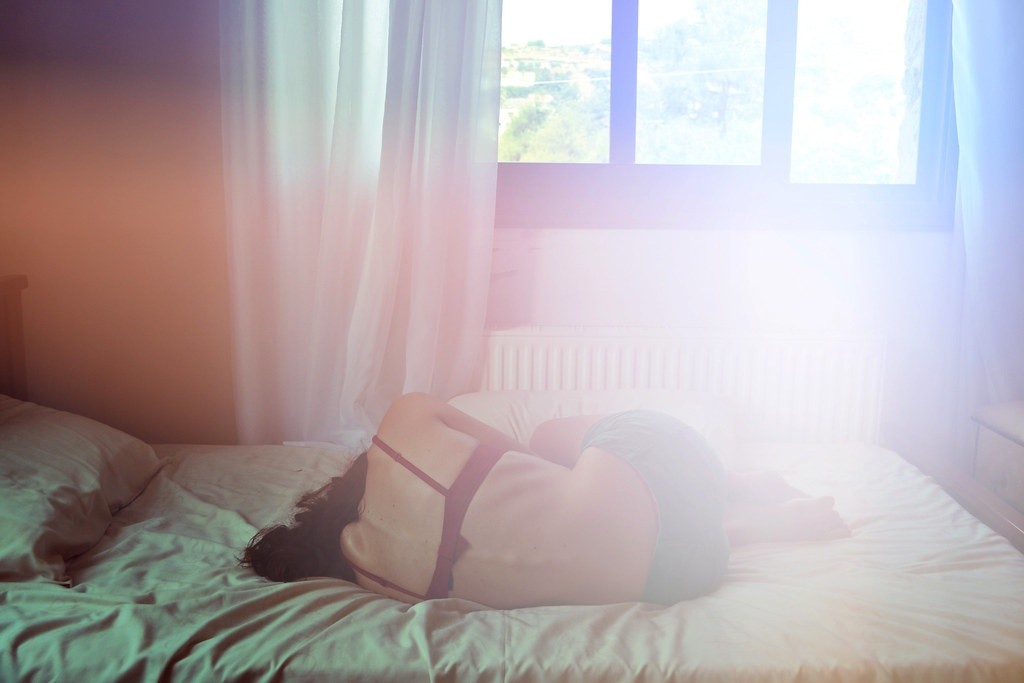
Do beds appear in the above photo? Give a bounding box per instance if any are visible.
[0,413,1024,683]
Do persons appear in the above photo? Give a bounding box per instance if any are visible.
[232,390,853,608]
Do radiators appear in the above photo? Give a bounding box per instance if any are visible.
[487,324,880,438]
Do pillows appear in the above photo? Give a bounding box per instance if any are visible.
[0,391,174,585]
